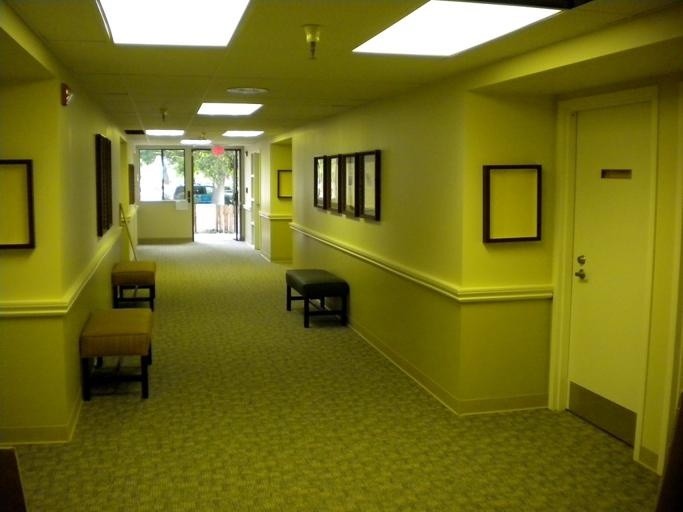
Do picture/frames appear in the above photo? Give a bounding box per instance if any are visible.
[0,156,36,251]
[93,132,112,237]
[275,168,293,199]
[312,148,383,223]
[482,160,544,244]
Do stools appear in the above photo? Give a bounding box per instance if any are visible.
[284,267,352,330]
[78,306,156,403]
[109,258,158,312]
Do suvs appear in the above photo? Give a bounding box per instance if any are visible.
[173,183,233,205]
